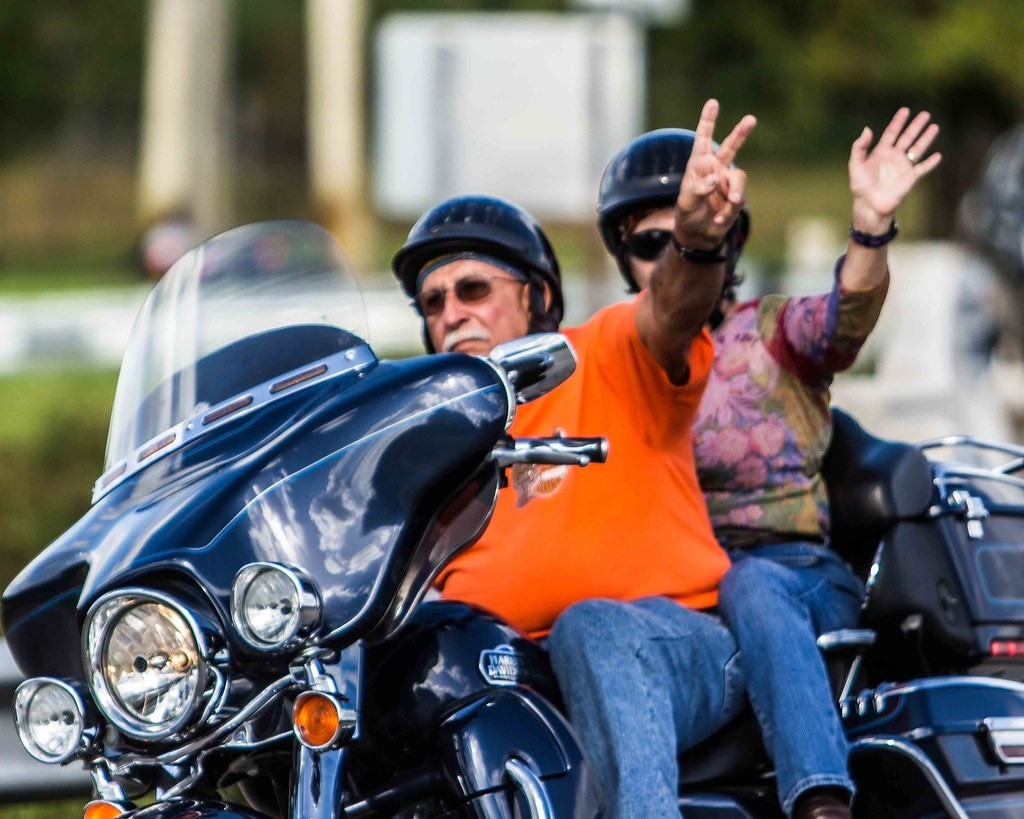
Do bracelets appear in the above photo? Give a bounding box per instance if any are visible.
[667,229,723,267]
[848,217,899,248]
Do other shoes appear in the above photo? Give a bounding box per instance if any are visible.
[794,787,851,819]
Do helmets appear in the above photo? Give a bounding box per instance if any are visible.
[390,195,564,335]
[594,127,751,253]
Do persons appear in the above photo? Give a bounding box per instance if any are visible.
[596,108,942,818]
[389,99,755,819]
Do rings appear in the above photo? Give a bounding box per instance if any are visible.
[904,150,917,164]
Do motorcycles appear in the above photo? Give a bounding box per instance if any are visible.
[0,222,1023,819]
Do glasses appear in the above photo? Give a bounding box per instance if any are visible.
[410,272,526,318]
[620,226,672,261]
